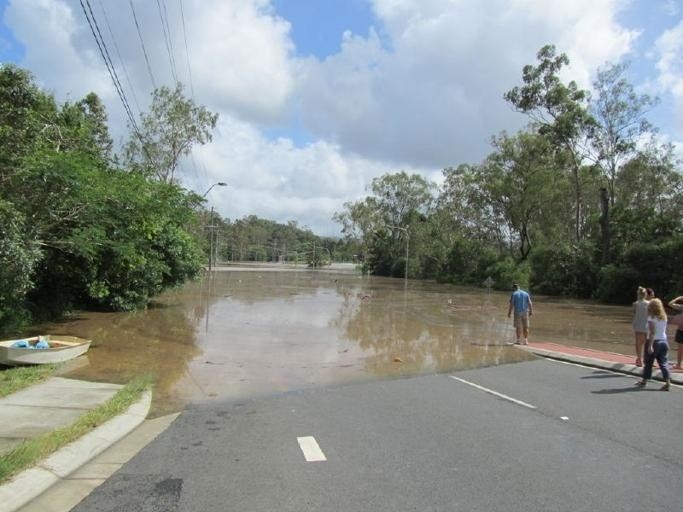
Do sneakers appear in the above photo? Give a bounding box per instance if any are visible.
[514,338,528,345]
[639,380,670,390]
[636,359,656,367]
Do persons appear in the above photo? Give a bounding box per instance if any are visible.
[634,297,671,391]
[667,295,682,372]
[631,286,649,368]
[506,283,533,344]
[645,288,661,370]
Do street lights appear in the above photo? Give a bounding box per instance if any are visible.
[193,183,227,209]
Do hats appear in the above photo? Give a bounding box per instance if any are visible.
[512,283,520,289]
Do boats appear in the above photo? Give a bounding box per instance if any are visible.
[0,335,92,365]
[517,342,683,384]
